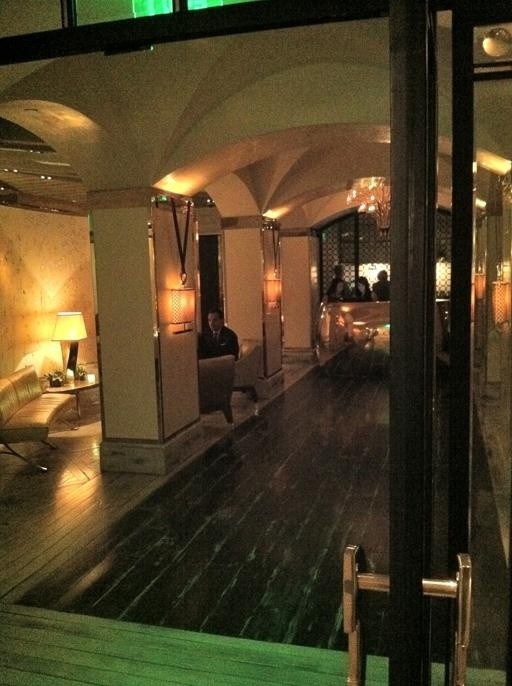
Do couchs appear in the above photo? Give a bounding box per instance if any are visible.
[0,365,76,471]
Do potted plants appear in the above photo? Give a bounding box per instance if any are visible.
[45,369,65,386]
[75,367,87,380]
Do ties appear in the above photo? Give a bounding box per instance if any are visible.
[213,333,217,343]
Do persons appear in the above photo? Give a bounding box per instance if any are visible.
[207,308,240,424]
[326,264,390,302]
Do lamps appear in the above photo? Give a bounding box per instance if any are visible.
[490,282,510,323]
[474,274,485,299]
[346,178,391,233]
[265,279,281,301]
[51,311,87,380]
[171,287,196,334]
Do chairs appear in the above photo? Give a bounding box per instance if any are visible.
[233,343,261,399]
[199,354,234,424]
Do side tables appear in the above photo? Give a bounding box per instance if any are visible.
[44,374,99,418]
